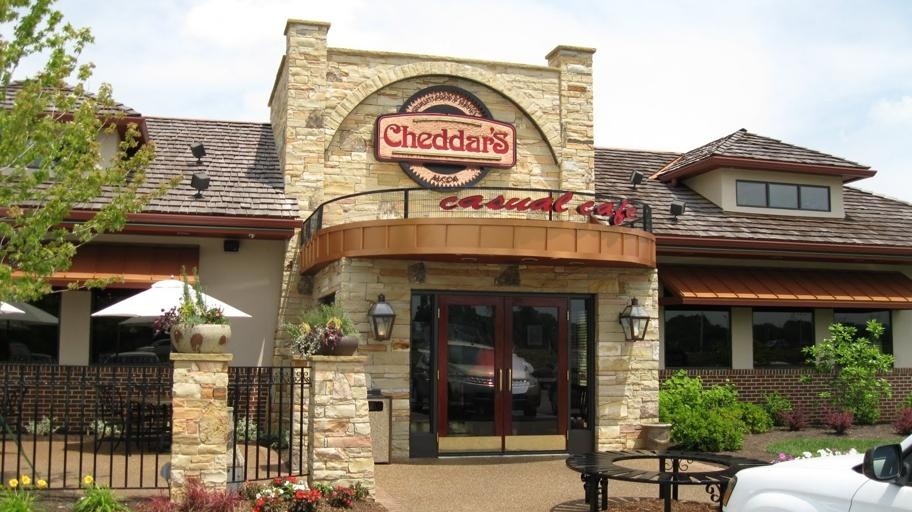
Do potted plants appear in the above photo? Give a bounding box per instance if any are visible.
[284,303,360,356]
[159,266,232,353]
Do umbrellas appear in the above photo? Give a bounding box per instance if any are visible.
[0,302,59,360]
[91,275,253,353]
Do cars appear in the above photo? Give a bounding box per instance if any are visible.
[413,339,556,418]
[103,340,170,365]
[721,432,912,512]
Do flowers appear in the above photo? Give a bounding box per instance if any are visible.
[0,475,114,511]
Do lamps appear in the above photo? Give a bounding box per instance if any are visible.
[365,294,396,341]
[618,296,650,344]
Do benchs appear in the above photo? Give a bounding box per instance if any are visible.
[565,450,774,512]
